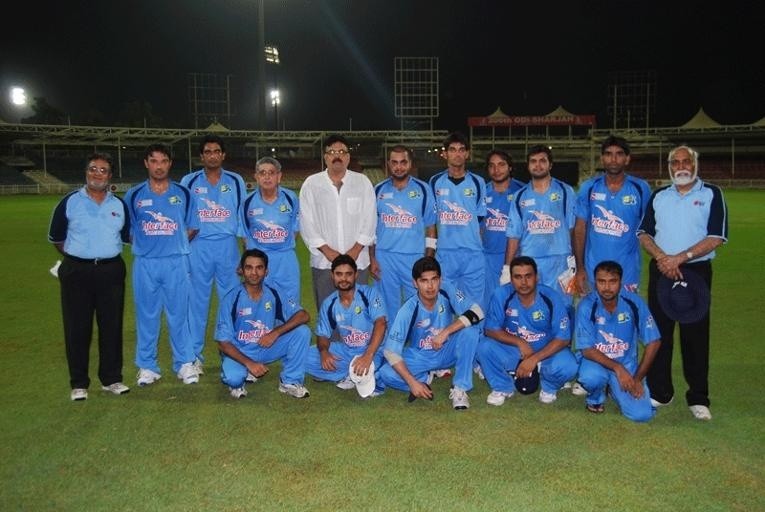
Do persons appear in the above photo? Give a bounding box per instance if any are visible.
[378,257,486,409]
[500,145,576,351]
[573,135,653,298]
[213,249,311,399]
[121,144,200,387]
[178,136,247,377]
[369,145,438,329]
[475,256,578,407]
[304,254,391,397]
[429,132,488,308]
[573,261,663,423]
[478,149,527,314]
[237,157,301,306]
[48,154,133,402]
[299,135,377,313]
[635,145,728,421]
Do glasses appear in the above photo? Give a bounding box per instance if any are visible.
[258,169,279,176]
[87,165,109,175]
[325,149,348,157]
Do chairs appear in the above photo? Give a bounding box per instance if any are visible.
[625,161,765,180]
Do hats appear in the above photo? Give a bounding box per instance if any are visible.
[655,270,711,324]
[349,355,376,398]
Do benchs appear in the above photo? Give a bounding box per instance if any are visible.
[0,155,385,189]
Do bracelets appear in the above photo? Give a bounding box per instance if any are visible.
[426,238,437,250]
[461,309,479,326]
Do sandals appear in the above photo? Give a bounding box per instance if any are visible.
[587,404,604,412]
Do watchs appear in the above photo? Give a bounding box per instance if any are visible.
[684,248,695,261]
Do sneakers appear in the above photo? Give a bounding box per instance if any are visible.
[649,395,674,407]
[229,385,247,399]
[176,362,200,385]
[71,388,88,401]
[136,368,163,387]
[486,391,514,406]
[194,357,204,375]
[539,382,589,404]
[246,372,257,382]
[688,404,711,421]
[278,377,310,399]
[102,382,130,394]
[427,366,485,409]
[336,376,355,390]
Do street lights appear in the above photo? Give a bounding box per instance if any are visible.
[264,43,280,131]
